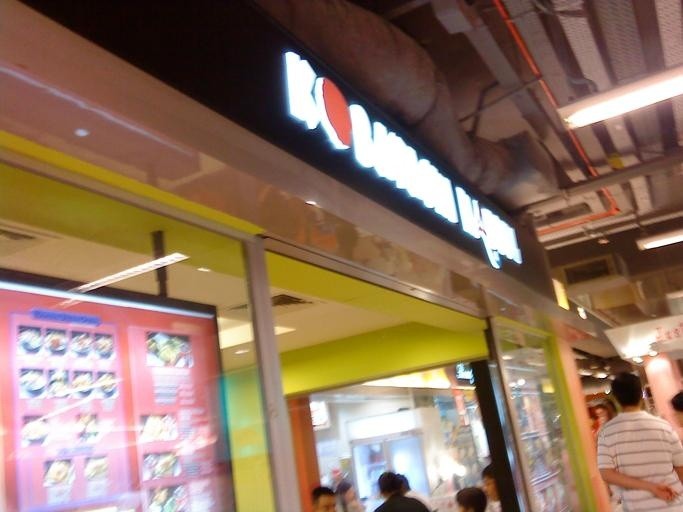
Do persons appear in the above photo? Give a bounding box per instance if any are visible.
[669,386,682,428]
[335,480,365,511]
[481,462,505,502]
[456,487,488,511]
[396,473,434,511]
[310,486,338,512]
[593,370,682,511]
[372,470,430,511]
[591,402,609,430]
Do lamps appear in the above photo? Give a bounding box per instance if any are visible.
[555,65,682,133]
[621,210,683,256]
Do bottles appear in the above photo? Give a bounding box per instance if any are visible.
[367,444,392,498]
[328,467,343,492]
[434,380,575,511]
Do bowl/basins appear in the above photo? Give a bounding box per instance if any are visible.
[19,332,196,510]
